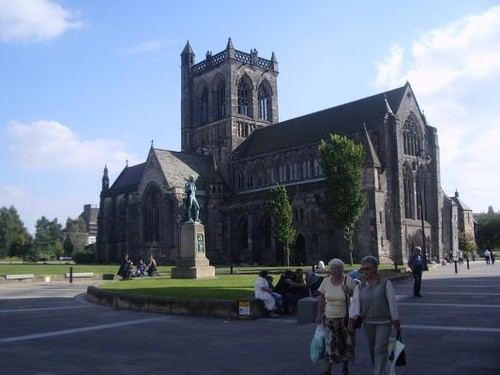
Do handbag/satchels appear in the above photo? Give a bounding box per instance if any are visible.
[344,276,363,329]
[311,323,325,364]
[391,327,406,366]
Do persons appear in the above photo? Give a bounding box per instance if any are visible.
[456,251,463,265]
[484,248,495,264]
[347,256,401,375]
[448,250,453,266]
[255,270,309,317]
[118,254,157,278]
[315,258,357,375]
[408,246,428,298]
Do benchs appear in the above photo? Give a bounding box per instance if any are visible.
[5,272,94,282]
[297,297,320,323]
